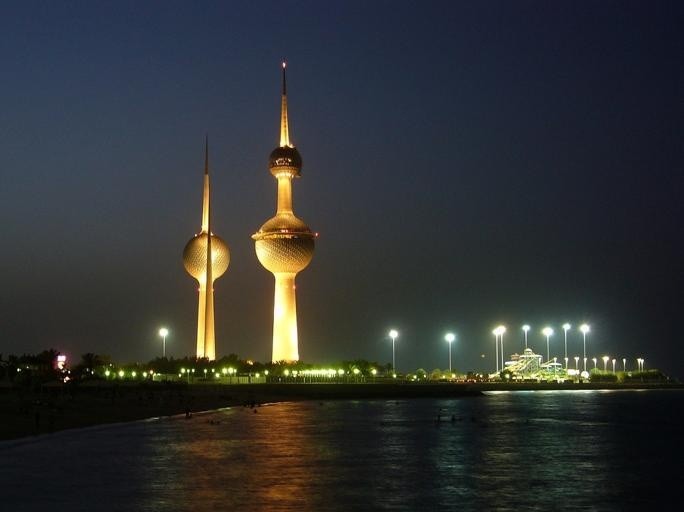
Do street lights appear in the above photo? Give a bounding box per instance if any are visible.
[387,330,399,376]
[542,327,553,368]
[444,332,455,373]
[579,324,590,357]
[492,324,505,373]
[158,327,168,358]
[552,355,645,375]
[521,325,531,349]
[562,323,571,358]
